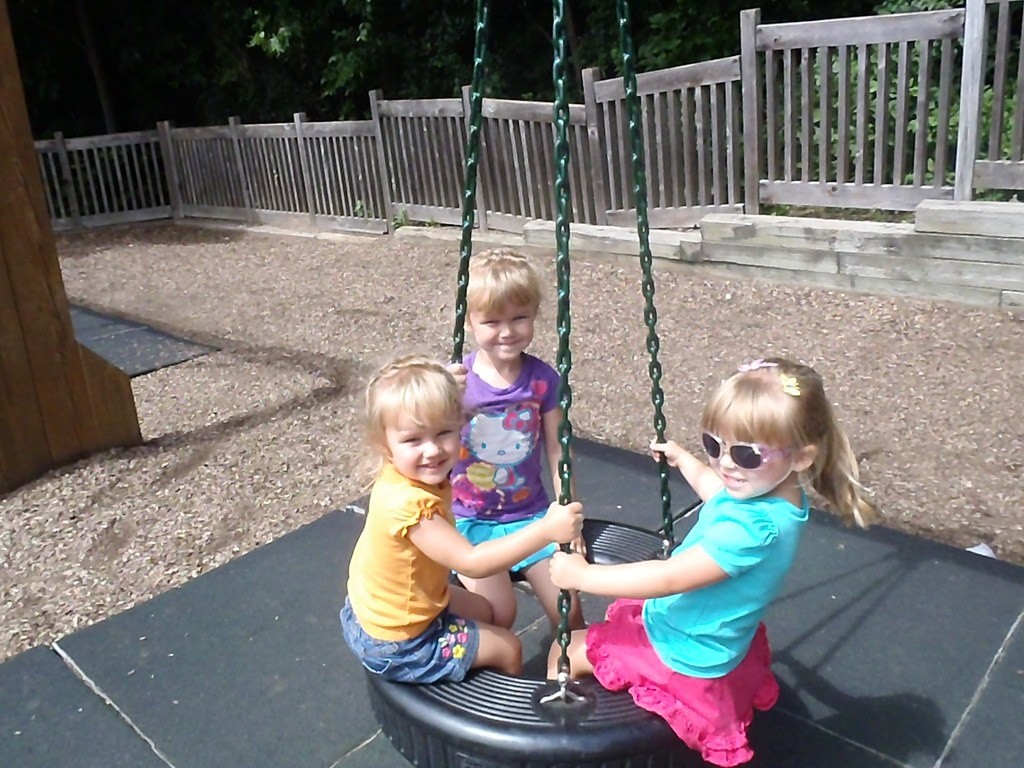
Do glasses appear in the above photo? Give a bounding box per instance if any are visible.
[700,428,800,471]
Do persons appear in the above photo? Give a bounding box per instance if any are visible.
[341,354,585,683]
[543,356,877,754]
[441,247,587,643]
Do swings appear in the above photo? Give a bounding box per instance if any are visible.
[354,0,716,768]
